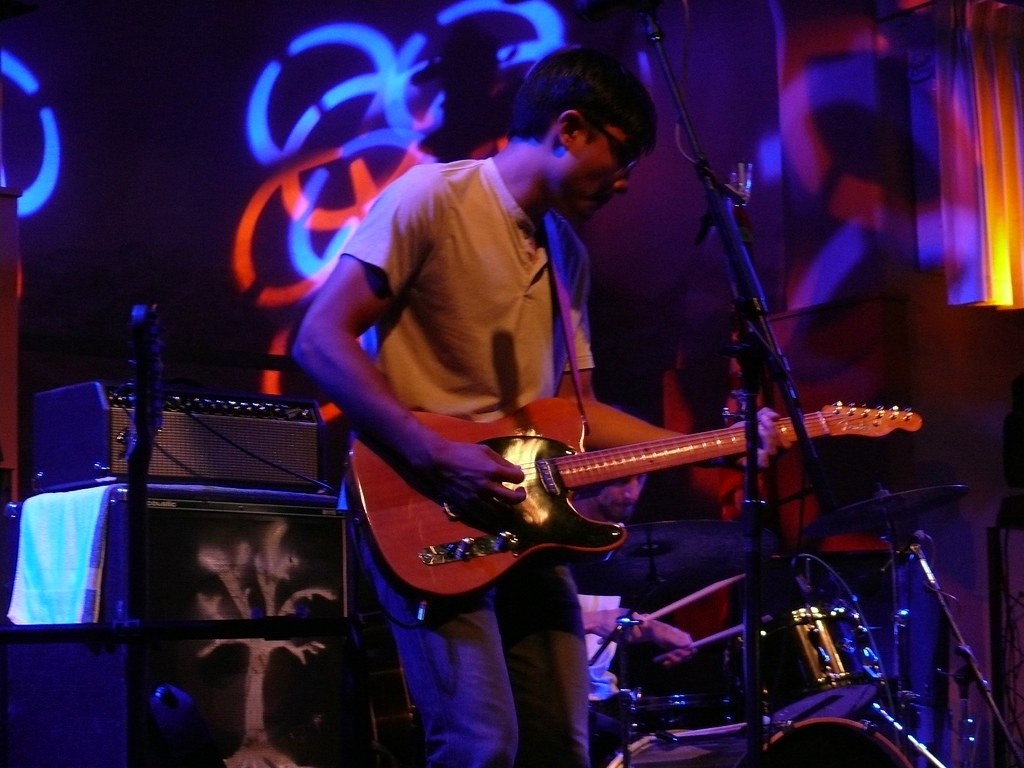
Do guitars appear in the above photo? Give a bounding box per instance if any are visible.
[341,397,927,622]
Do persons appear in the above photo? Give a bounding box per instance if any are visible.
[565,471,696,768]
[293,44,791,768]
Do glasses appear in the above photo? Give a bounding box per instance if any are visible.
[570,105,638,177]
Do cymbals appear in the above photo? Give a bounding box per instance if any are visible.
[575,518,778,600]
[801,482,970,541]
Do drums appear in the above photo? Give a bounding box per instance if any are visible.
[605,721,751,768]
[756,718,913,768]
[633,690,741,730]
[716,597,884,728]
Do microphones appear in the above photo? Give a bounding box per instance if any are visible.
[881,530,925,573]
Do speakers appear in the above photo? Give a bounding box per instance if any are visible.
[7,480,352,768]
